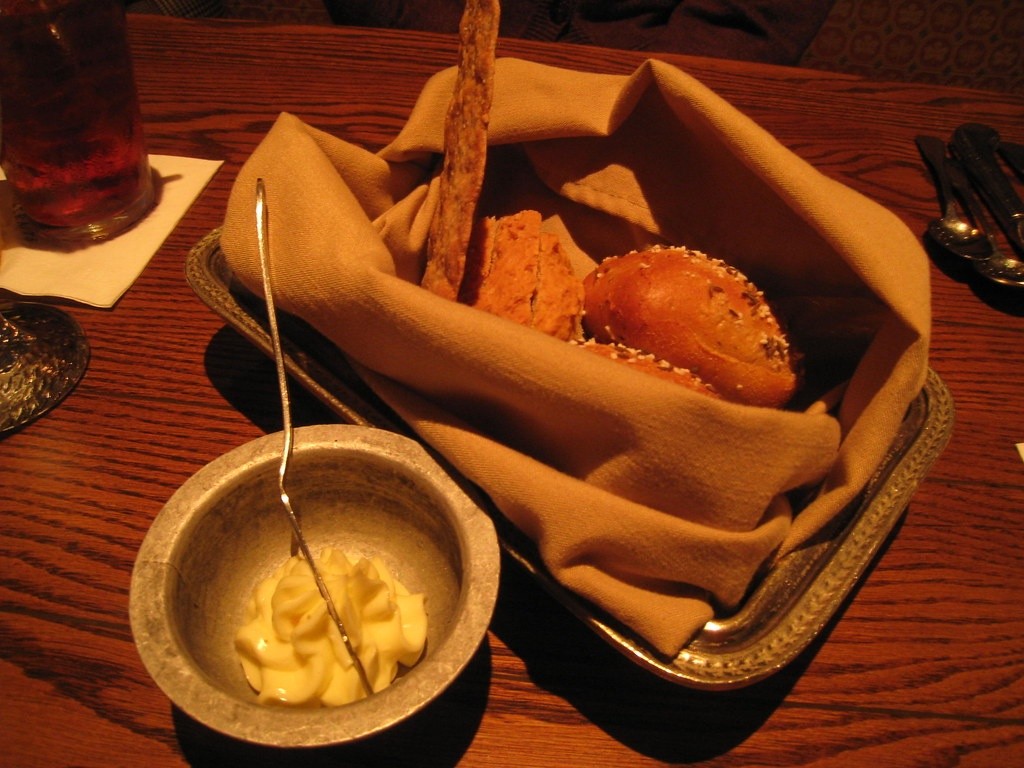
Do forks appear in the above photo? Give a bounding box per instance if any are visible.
[254,180,374,691]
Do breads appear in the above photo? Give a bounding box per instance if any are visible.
[463,209,801,413]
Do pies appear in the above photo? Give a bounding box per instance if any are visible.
[421,0,501,301]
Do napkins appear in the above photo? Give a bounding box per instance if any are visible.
[1,148,226,311]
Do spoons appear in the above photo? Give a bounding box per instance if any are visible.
[915,136,1024,287]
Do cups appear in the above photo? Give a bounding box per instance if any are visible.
[0,0,154,243]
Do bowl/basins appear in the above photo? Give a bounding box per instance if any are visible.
[129,424,501,748]
[184,223,955,690]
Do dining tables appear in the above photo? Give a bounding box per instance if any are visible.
[1,12,1024,768]
